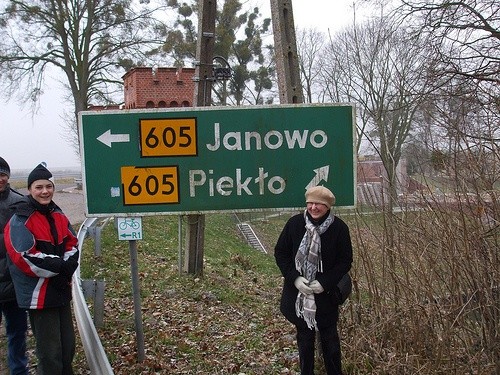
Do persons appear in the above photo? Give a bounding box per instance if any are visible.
[274,185,354,375]
[0,156,24,375]
[3,162,80,375]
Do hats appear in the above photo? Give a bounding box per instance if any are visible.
[0,157,10,178]
[28,164,54,190]
[304,185,335,209]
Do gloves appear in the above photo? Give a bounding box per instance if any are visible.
[295,276,323,297]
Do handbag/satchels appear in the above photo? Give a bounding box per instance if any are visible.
[336,272,352,304]
